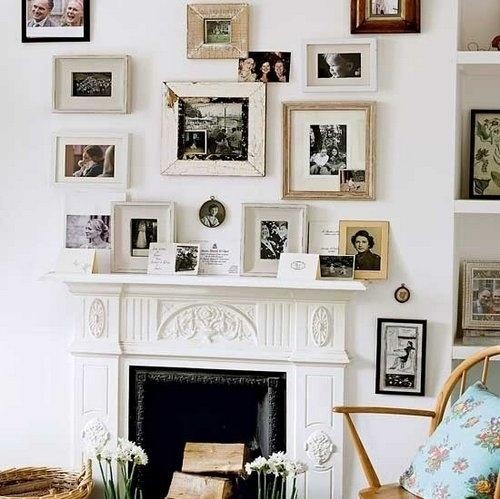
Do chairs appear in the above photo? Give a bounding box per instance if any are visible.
[329,342,500,499]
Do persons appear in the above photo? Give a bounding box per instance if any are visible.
[27,0,57,28]
[311,125,366,193]
[472,286,496,321]
[72,144,104,177]
[273,58,288,84]
[236,55,257,82]
[207,125,243,153]
[350,230,381,271]
[59,0,85,27]
[74,217,111,250]
[260,221,288,259]
[176,246,195,271]
[388,340,416,370]
[337,264,348,275]
[259,60,271,82]
[134,221,149,249]
[319,53,361,77]
[329,263,336,274]
[199,203,222,228]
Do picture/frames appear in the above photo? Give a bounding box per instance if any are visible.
[239,201,304,275]
[108,197,178,276]
[459,256,500,337]
[54,133,137,195]
[348,2,421,34]
[185,2,250,60]
[469,108,500,200]
[160,80,266,175]
[281,99,376,202]
[49,52,131,115]
[336,219,388,281]
[59,210,113,250]
[300,37,376,92]
[18,1,93,43]
[373,316,428,398]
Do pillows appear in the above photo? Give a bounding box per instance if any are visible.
[399,376,500,499]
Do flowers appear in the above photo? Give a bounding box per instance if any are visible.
[242,450,309,499]
[93,428,151,499]
